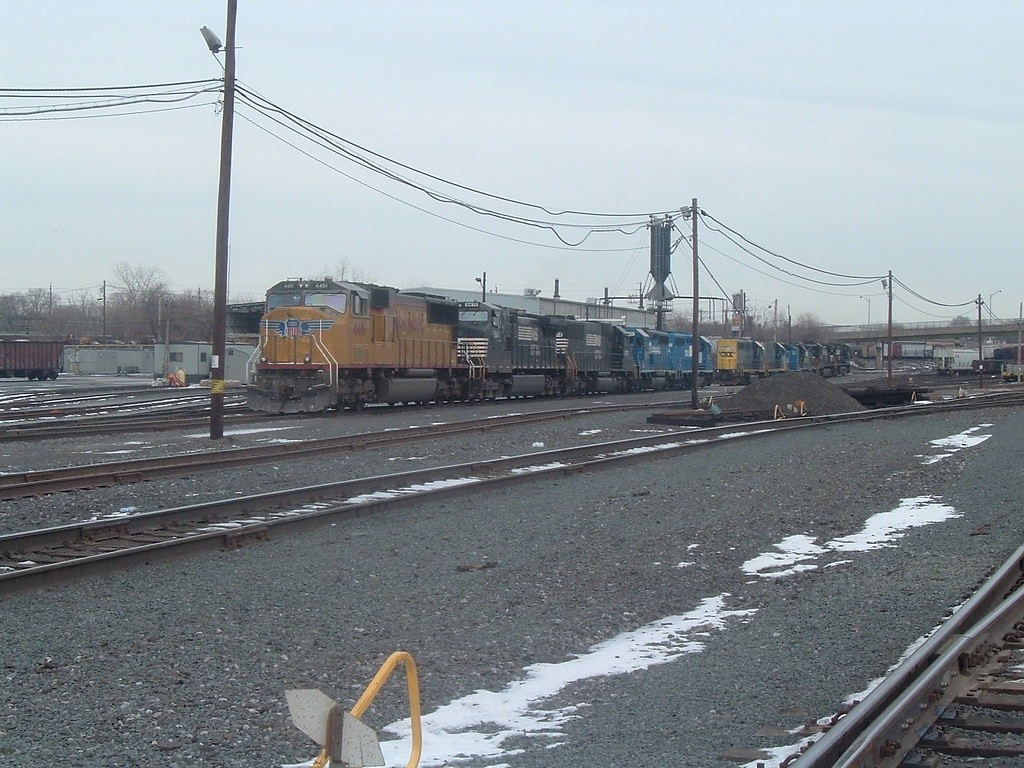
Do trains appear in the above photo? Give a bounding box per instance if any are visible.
[246,275,851,417]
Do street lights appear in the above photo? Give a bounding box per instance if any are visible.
[767,298,778,343]
[859,295,871,325]
[680,197,700,410]
[199,0,238,440]
[881,269,893,378]
[475,272,486,302]
[990,290,1001,324]
